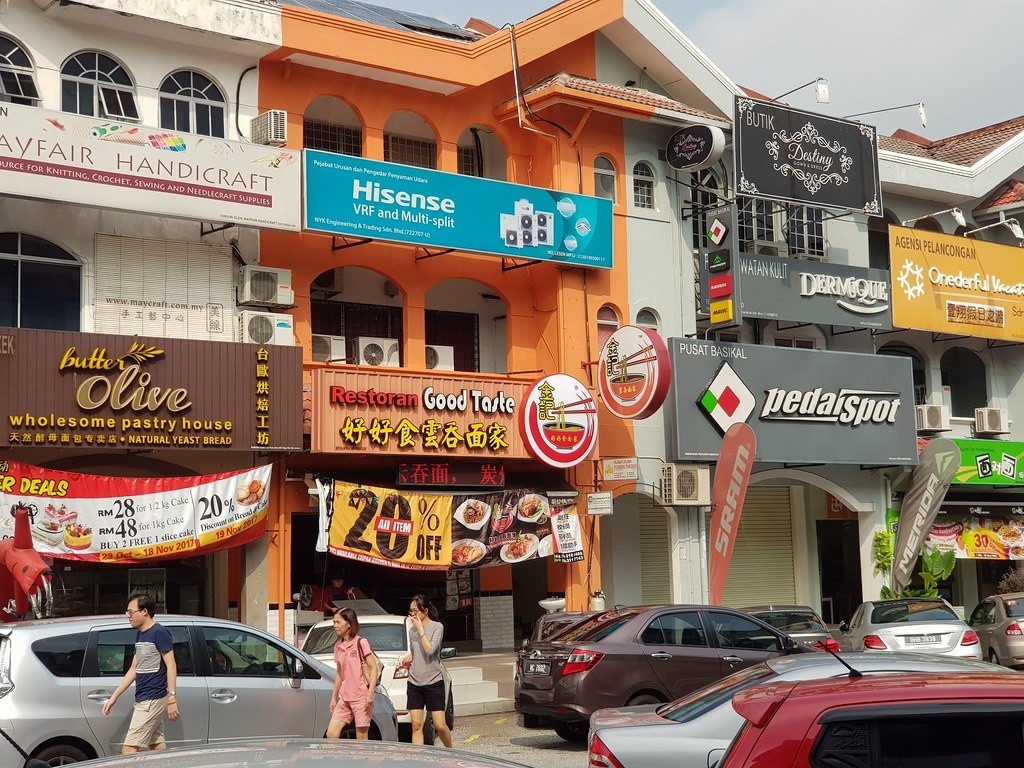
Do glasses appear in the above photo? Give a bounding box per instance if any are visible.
[409,608,423,615]
[126,609,142,618]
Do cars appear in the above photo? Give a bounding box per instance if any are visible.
[300,613,456,745]
[586,648,1019,768]
[715,602,839,654]
[522,613,599,643]
[0,614,398,768]
[839,596,983,662]
[966,591,1024,669]
[39,738,529,767]
[513,603,822,740]
[715,673,1023,768]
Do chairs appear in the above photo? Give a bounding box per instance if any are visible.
[682,627,700,645]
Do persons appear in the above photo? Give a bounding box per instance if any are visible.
[326,607,378,740]
[102,593,180,755]
[397,596,452,748]
[323,573,353,616]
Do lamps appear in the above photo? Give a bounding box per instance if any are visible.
[963,218,1024,239]
[903,207,967,227]
[843,103,927,127]
[771,76,829,103]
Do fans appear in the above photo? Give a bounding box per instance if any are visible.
[293,585,313,648]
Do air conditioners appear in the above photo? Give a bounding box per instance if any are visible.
[915,404,950,430]
[309,266,344,294]
[355,336,401,369]
[974,407,1010,433]
[250,109,289,146]
[237,265,292,307]
[311,334,347,364]
[660,463,711,504]
[238,310,294,345]
[424,344,455,372]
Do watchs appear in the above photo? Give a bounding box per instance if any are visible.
[167,688,176,696]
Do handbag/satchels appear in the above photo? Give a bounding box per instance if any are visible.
[356,637,384,688]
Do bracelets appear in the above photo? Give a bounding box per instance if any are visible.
[167,696,177,704]
[420,632,425,639]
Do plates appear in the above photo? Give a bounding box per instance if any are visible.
[34,519,63,533]
[452,494,554,566]
[990,519,1024,557]
